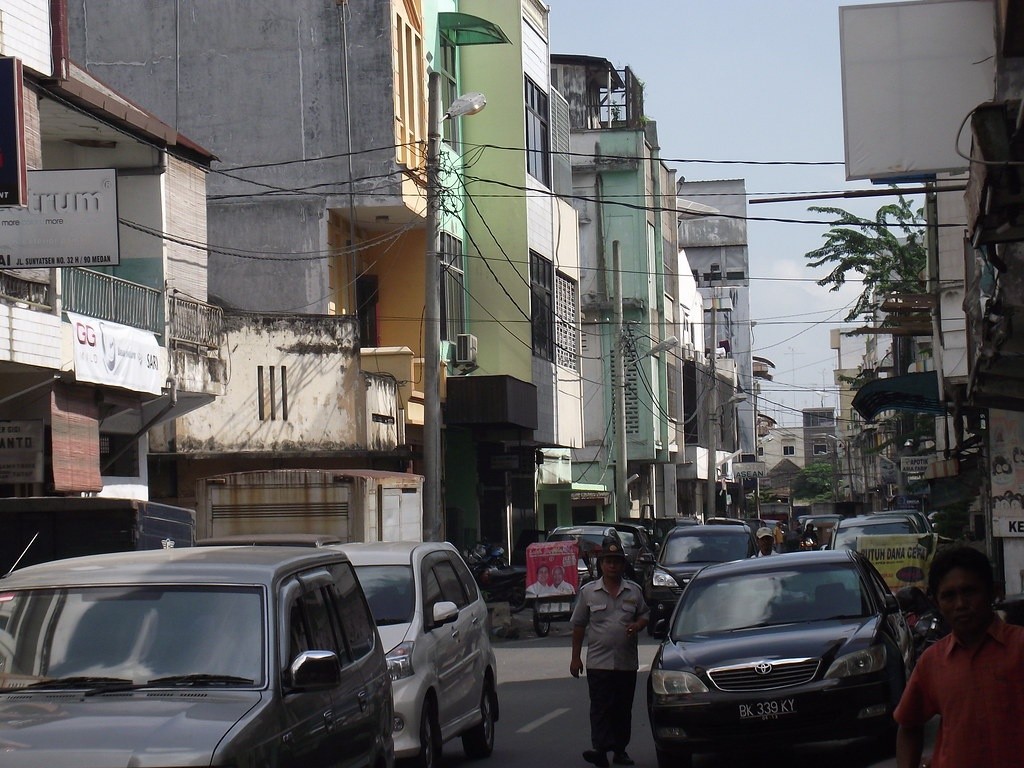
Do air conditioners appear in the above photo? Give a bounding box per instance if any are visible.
[456,334,478,367]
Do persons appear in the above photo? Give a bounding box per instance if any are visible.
[527,565,575,597]
[751,518,818,561]
[891,547,1024,768]
[570,544,648,768]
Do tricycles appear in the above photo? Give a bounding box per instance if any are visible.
[524,542,594,638]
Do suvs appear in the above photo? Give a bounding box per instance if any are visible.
[0,547,392,768]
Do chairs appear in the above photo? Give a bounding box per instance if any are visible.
[814,582,850,613]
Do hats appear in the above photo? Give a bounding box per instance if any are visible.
[756,527,773,539]
[599,542,626,558]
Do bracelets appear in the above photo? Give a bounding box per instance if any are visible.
[638,622,645,629]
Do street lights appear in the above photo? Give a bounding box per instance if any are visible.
[755,436,775,520]
[612,335,681,525]
[828,435,856,516]
[420,65,500,557]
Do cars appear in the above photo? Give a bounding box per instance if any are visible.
[652,516,791,554]
[798,510,943,640]
[586,521,656,587]
[639,525,759,637]
[645,551,929,768]
[547,526,622,589]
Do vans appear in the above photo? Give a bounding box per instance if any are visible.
[314,541,501,768]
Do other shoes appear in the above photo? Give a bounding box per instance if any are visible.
[613,752,635,766]
[582,750,610,768]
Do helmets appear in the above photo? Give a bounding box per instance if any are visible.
[807,523,814,530]
